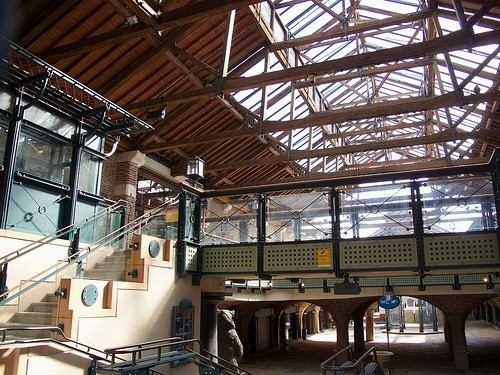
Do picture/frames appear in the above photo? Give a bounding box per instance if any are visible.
[171,306,195,367]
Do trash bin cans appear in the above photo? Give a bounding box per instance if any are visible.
[302,329,307,340]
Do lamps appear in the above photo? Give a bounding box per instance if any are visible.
[186,154,205,181]
[298,283,305,293]
[453,276,461,290]
[386,277,393,292]
[418,280,425,291]
[486,274,495,289]
